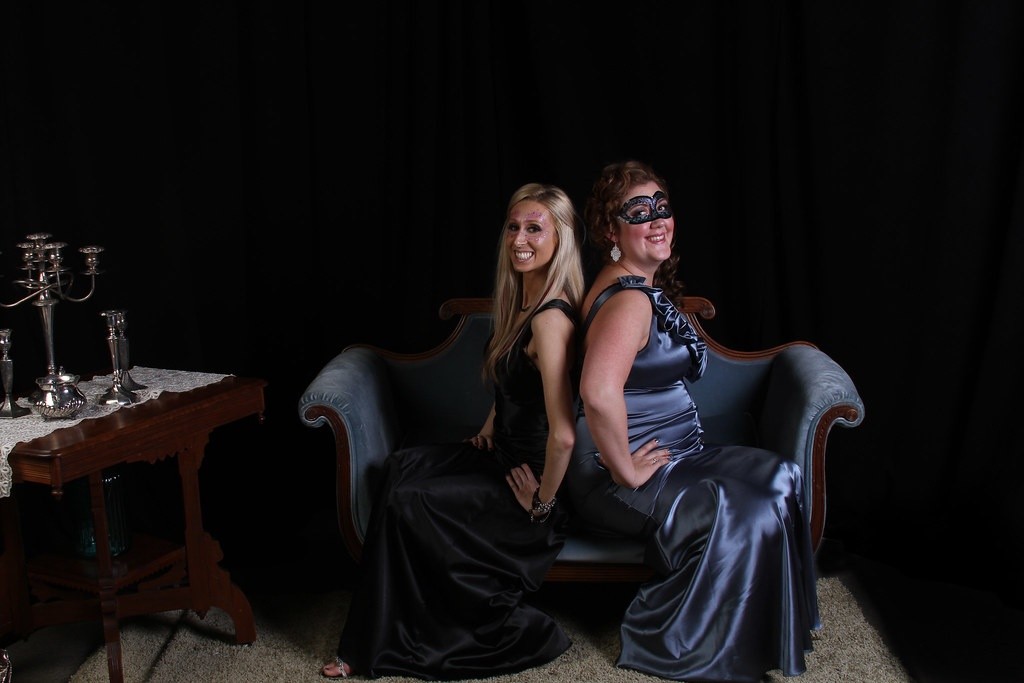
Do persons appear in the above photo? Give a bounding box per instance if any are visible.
[562,159,822,681]
[320,178,585,680]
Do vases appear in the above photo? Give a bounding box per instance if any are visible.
[78,468,127,559]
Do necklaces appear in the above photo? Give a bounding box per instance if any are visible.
[519,304,532,313]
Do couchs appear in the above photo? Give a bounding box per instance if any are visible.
[297,292,866,585]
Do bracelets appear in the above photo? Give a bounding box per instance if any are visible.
[528,485,556,524]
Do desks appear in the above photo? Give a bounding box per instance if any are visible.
[1,364,271,683]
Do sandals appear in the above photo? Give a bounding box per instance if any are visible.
[324,655,355,679]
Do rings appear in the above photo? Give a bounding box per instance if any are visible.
[477,434,482,439]
[651,456,658,464]
